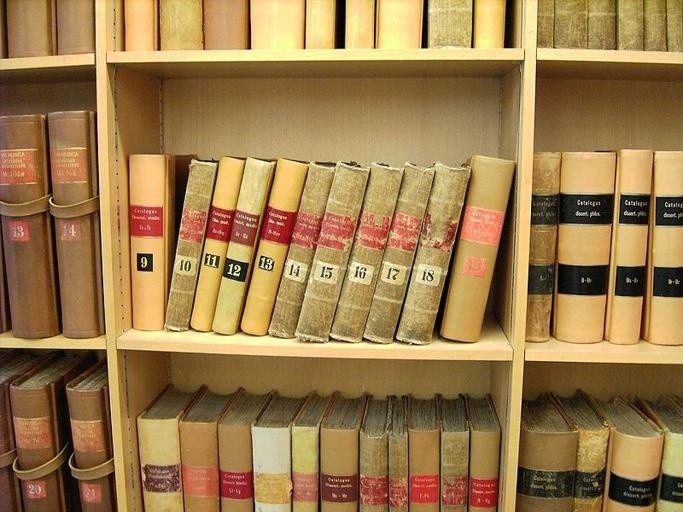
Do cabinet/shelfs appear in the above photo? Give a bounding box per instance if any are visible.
[0,0,683,512]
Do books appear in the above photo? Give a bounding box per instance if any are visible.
[523,146,683,347]
[133,381,502,512]
[0,108,106,339]
[538,1,683,54]
[122,1,507,49]
[513,387,683,512]
[127,151,516,346]
[0,1,96,60]
[0,349,118,512]
